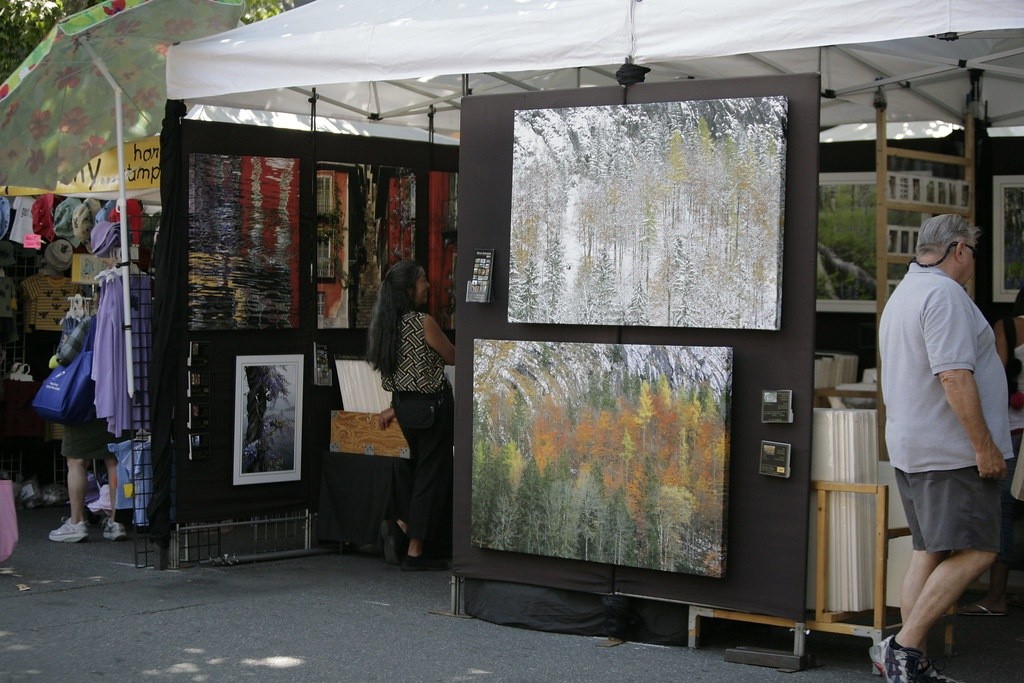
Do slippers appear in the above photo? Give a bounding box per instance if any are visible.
[958,602,1005,616]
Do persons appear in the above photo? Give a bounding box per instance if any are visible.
[957,285,1024,615]
[367,261,456,572]
[868,213,1015,683]
[48,285,128,544]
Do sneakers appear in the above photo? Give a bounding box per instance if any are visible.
[48,516,90,542]
[868,634,927,683]
[102,518,127,540]
[917,660,957,683]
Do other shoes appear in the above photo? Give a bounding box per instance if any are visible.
[401,548,449,570]
[381,519,407,567]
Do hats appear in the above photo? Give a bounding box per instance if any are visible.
[0,194,124,272]
[8,466,121,516]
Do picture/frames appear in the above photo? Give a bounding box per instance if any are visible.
[232,353,304,487]
[817,171,935,312]
[993,173,1024,303]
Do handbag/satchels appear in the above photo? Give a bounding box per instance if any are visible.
[397,399,436,431]
[32,314,93,425]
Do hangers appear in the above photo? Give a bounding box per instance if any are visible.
[23,246,159,326]
[128,429,152,446]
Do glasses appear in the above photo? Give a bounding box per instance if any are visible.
[949,241,980,259]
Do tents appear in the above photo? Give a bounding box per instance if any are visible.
[145,0,1024,646]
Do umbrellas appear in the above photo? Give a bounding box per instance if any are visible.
[0,0,246,398]
